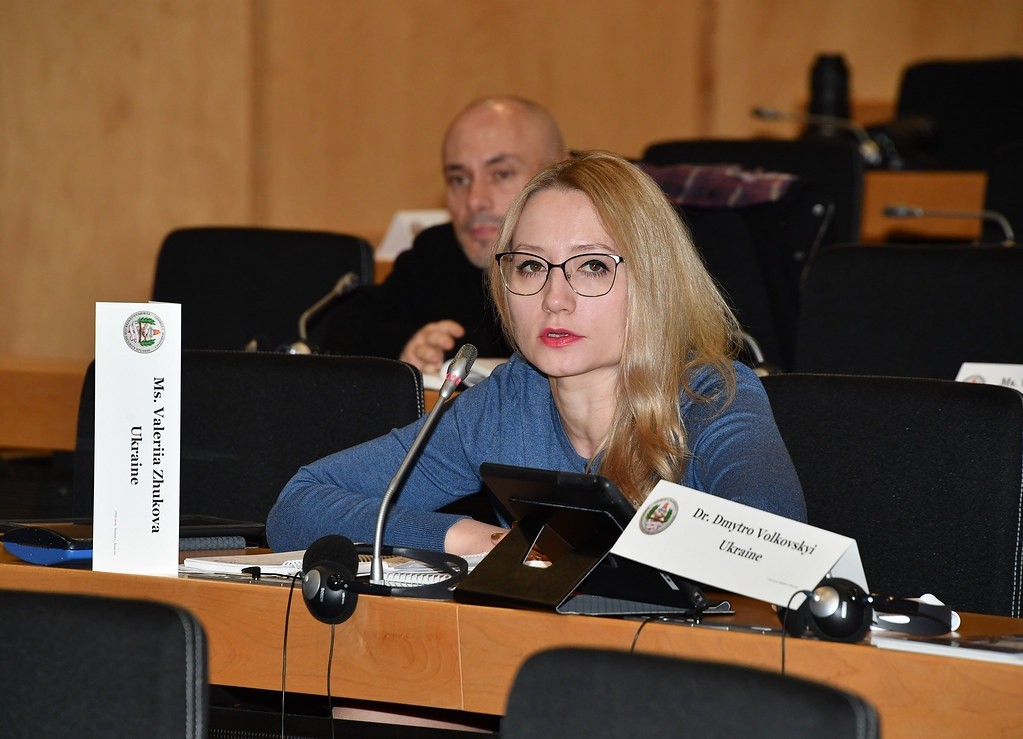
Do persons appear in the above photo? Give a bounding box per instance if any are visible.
[309,93,565,374]
[262,150,809,558]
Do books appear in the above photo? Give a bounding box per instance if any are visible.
[184,550,371,574]
[384,553,487,587]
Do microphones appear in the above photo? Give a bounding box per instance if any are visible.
[370,344,478,585]
[751,103,903,170]
[882,205,1014,240]
[299,271,359,340]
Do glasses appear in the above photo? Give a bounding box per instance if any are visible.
[494,251,625,298]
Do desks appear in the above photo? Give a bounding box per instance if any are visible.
[0,526,1023,739]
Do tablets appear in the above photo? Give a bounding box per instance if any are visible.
[478,462,709,611]
[0,515,266,548]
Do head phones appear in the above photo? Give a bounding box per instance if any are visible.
[777,577,952,642]
[301,535,468,624]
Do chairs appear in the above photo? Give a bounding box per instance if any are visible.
[0,54,1023,739]
